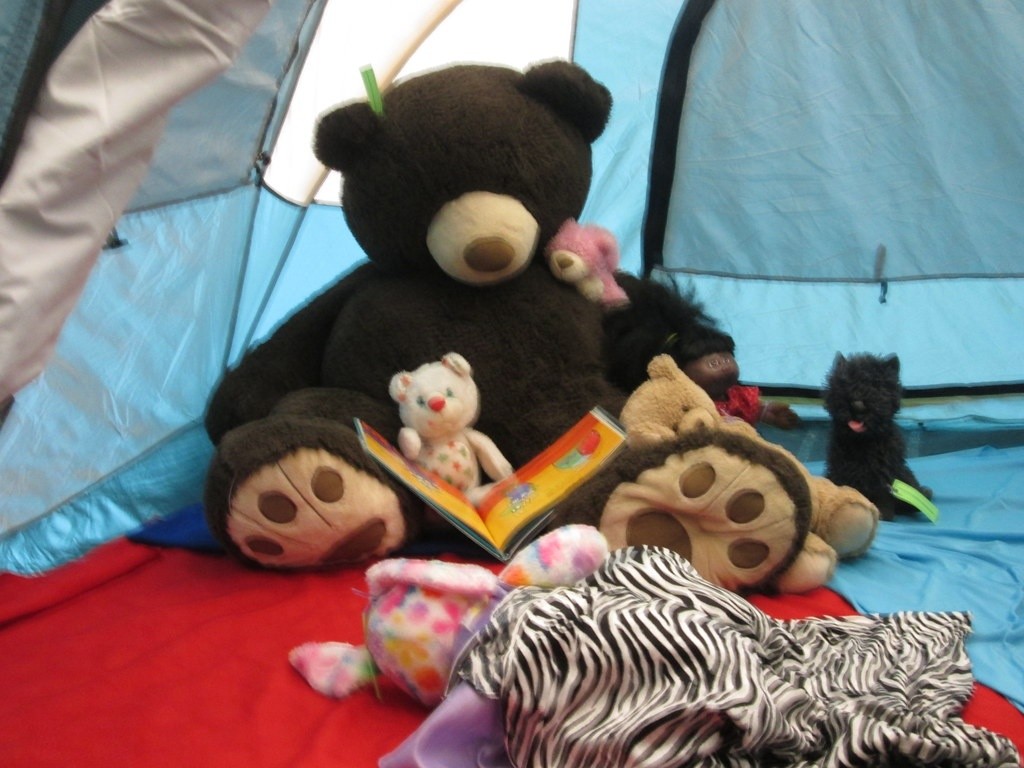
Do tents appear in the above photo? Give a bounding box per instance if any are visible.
[1,0,1024,767]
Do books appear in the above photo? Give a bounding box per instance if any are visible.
[353,408,629,563]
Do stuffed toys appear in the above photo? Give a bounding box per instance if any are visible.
[287,525,612,704]
[545,217,630,309]
[389,353,514,503]
[614,353,879,594]
[822,353,933,518]
[202,62,815,593]
